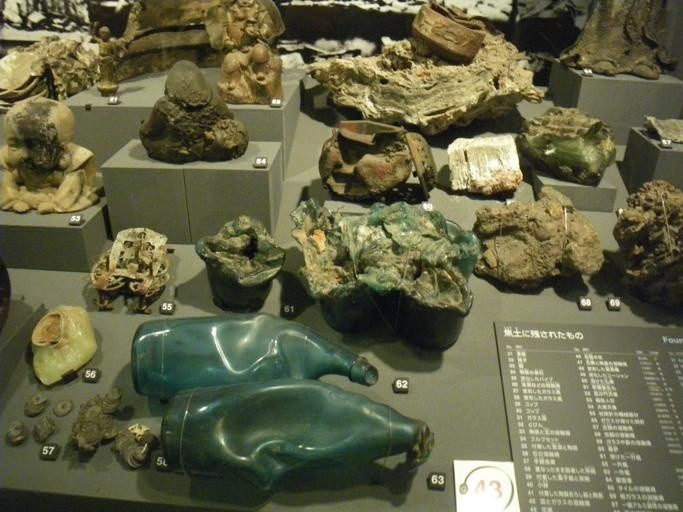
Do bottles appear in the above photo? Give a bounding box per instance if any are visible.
[130,312,379,403]
[160,378,436,497]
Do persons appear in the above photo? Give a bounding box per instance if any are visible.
[96,26,119,87]
[0,95,99,214]
[560,0,679,78]
[221,0,273,89]
[138,60,233,164]
